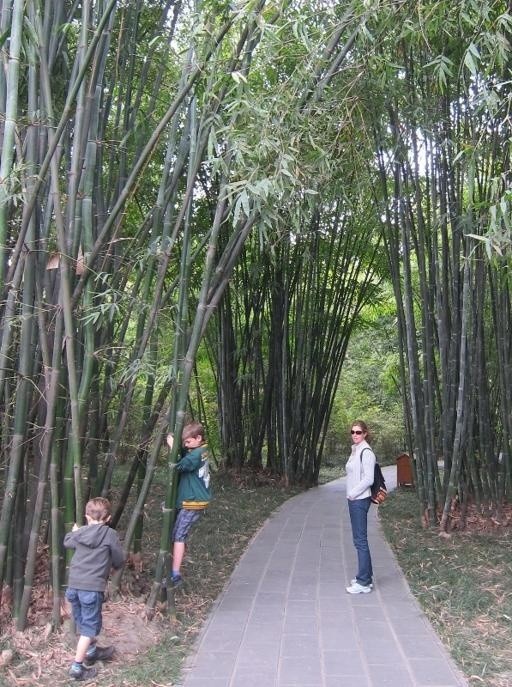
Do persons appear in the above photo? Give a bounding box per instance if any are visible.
[63,497,124,680]
[161,422,211,591]
[344,419,376,594]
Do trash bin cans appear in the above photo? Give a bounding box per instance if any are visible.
[396,452,417,491]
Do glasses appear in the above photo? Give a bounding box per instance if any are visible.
[351,430,363,435]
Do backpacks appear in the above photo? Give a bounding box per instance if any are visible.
[360,448,388,504]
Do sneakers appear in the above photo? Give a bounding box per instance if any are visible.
[162,572,183,590]
[69,667,98,681]
[345,576,373,594]
[84,645,115,666]
